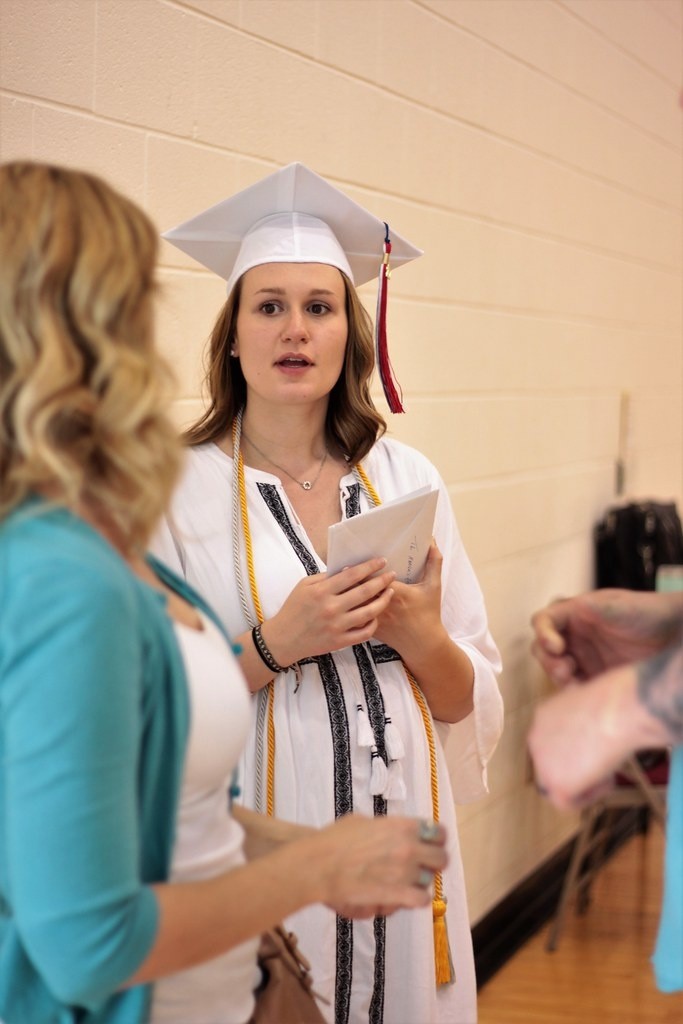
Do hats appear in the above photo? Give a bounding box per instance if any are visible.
[162,161,423,410]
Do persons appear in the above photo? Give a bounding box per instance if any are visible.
[0,160,452,1024]
[145,162,506,1024]
[530,587,683,691]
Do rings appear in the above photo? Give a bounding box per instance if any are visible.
[415,818,439,841]
[417,869,432,887]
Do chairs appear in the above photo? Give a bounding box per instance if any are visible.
[542,744,669,952]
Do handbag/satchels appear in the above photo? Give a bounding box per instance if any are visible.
[246,925,327,1024]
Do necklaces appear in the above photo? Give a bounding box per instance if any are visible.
[241,432,330,490]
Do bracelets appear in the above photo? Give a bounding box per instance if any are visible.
[251,624,289,675]
